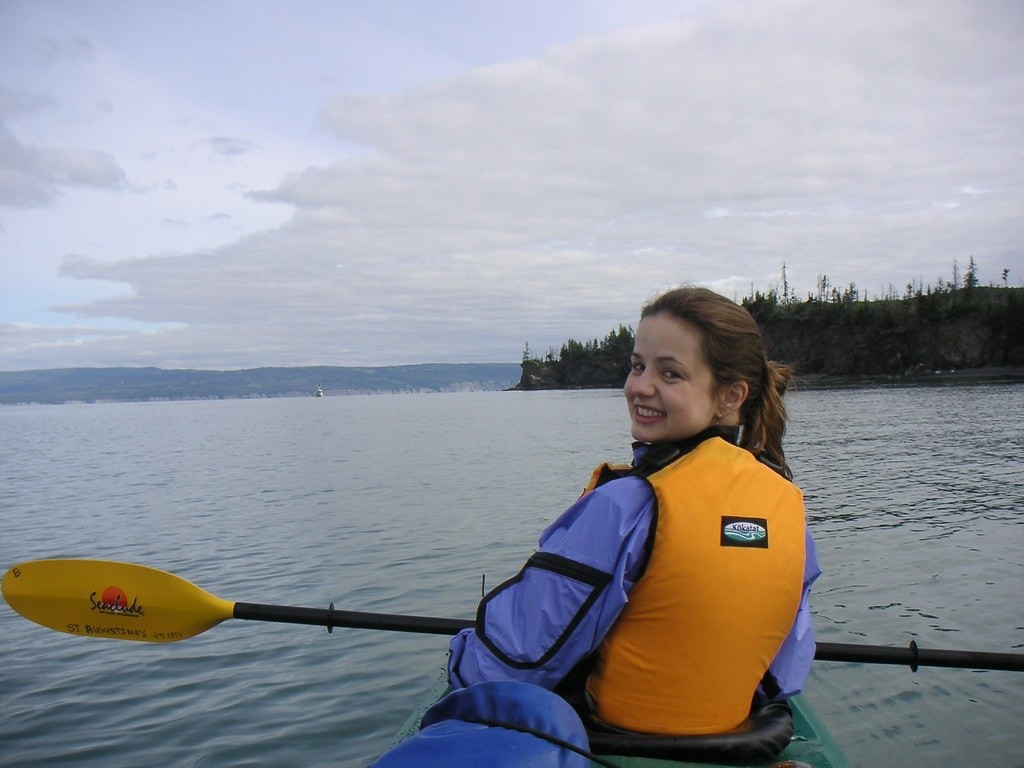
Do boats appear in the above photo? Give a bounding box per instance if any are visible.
[316,384,323,395]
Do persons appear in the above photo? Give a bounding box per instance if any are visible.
[447,288,821,736]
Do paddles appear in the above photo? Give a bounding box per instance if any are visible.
[2,558,1024,673]
